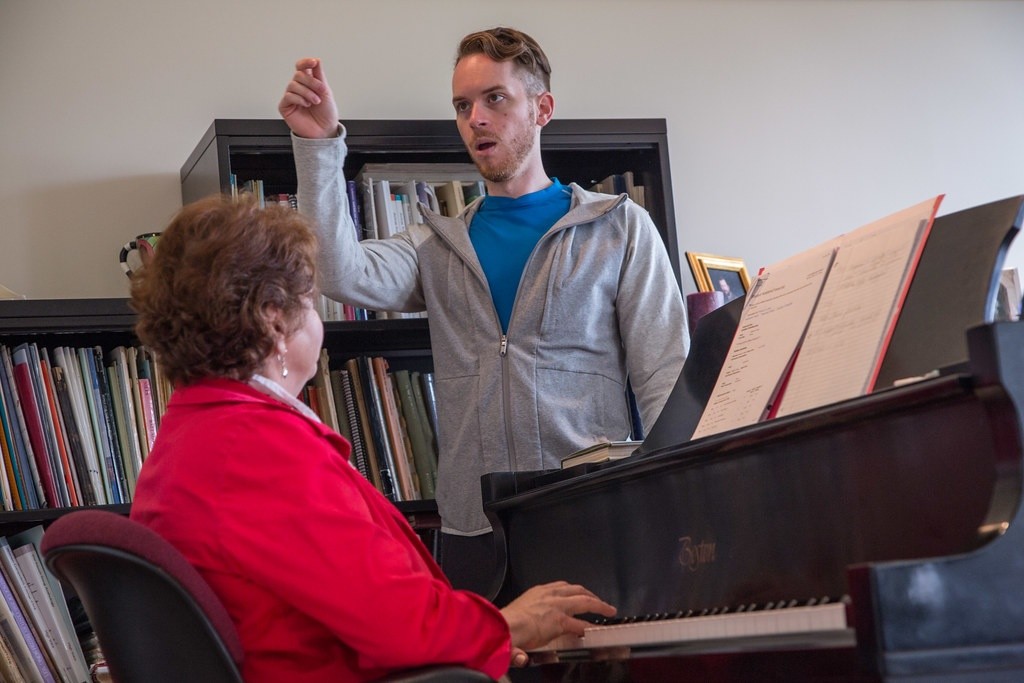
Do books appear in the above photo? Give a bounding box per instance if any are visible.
[230,172,484,322]
[589,170,645,211]
[0,342,174,511]
[296,348,439,502]
[560,440,643,470]
[0,525,113,683]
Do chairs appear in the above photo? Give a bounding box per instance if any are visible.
[38,510,246,683]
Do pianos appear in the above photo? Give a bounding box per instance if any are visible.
[483,196,1024,683]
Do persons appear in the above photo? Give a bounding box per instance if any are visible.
[122,193,616,683]
[717,274,739,305]
[278,28,690,603]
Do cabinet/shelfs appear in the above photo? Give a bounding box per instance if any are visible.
[179,117,682,565]
[0,298,212,683]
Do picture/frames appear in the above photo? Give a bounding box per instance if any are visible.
[685,251,753,307]
